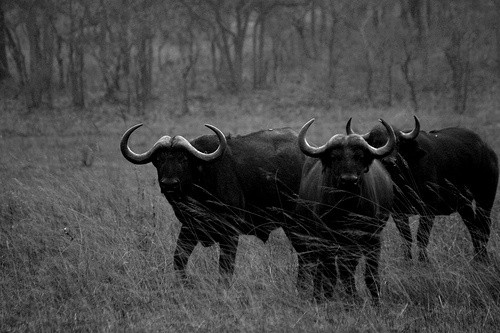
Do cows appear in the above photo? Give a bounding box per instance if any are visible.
[119,116,500,306]
